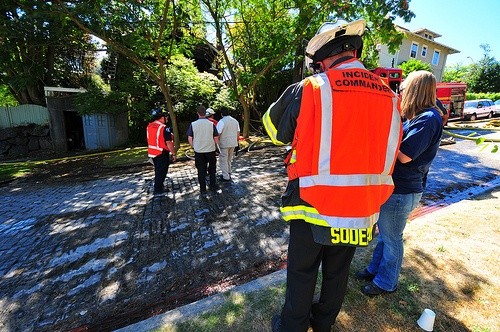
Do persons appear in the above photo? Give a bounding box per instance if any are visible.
[262,18,402,332]
[146,108,177,192]
[354,70,444,296]
[422,98,448,189]
[187,106,240,193]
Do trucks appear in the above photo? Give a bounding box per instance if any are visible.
[370,66,469,123]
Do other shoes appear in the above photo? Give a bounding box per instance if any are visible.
[154,185,170,193]
[216,173,232,183]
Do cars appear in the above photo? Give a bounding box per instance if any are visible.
[494,99,500,117]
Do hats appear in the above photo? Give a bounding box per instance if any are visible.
[149,106,169,122]
[206,107,216,116]
[305,18,367,73]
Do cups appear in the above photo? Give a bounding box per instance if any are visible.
[417,308,436,331]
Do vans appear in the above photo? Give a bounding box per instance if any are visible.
[462,99,495,121]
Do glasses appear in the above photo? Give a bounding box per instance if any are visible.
[398,84,405,91]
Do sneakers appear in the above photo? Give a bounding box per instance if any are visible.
[355,267,374,280]
[310,302,319,320]
[270,314,281,331]
[361,282,396,296]
